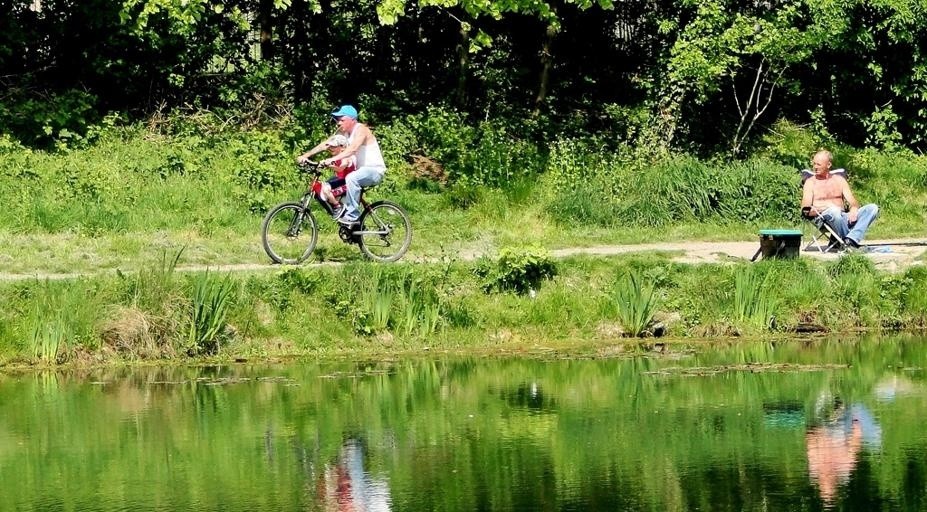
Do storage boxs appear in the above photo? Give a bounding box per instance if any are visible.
[759,229,804,259]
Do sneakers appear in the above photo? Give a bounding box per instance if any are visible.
[331,203,346,221]
[338,217,361,225]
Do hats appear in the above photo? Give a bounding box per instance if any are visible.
[325,134,346,147]
[331,105,358,120]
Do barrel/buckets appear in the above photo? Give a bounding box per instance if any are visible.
[752,227,803,260]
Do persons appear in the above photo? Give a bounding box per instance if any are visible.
[302,134,356,223]
[799,149,879,253]
[294,104,389,245]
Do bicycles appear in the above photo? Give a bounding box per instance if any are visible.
[261,158,412,265]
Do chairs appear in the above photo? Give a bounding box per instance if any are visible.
[801,168,849,254]
